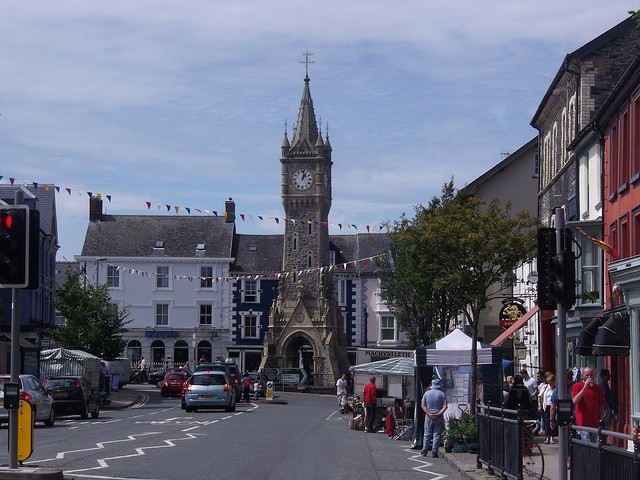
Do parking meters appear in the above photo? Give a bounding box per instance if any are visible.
[557,399,574,426]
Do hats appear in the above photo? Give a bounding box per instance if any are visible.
[430,379,443,389]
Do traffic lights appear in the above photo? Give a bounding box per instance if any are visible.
[550,252,575,306]
[0,204,29,289]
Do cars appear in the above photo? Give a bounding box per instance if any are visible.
[185,370,236,413]
[43,376,100,420]
[161,372,189,397]
[147,368,192,388]
[181,376,195,412]
[240,372,271,397]
[0,374,55,425]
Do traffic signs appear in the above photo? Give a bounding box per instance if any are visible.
[281,368,308,385]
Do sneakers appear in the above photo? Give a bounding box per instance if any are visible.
[432,452,438,457]
[365,428,374,432]
[420,450,426,456]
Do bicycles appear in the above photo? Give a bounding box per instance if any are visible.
[514,409,543,479]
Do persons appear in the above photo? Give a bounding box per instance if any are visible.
[421,378,447,458]
[139,356,147,382]
[507,375,529,410]
[537,371,544,385]
[253,380,260,400]
[336,372,348,411]
[538,374,558,444]
[598,369,616,430]
[571,367,607,443]
[362,376,377,433]
[538,372,552,433]
[506,376,515,391]
[243,372,251,403]
[550,370,575,470]
[519,368,538,411]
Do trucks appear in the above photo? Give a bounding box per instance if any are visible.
[100,357,131,389]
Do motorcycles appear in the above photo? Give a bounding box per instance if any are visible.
[131,370,146,384]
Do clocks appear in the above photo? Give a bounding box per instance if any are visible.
[291,168,313,191]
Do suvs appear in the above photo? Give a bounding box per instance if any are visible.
[196,363,236,401]
[219,362,242,402]
